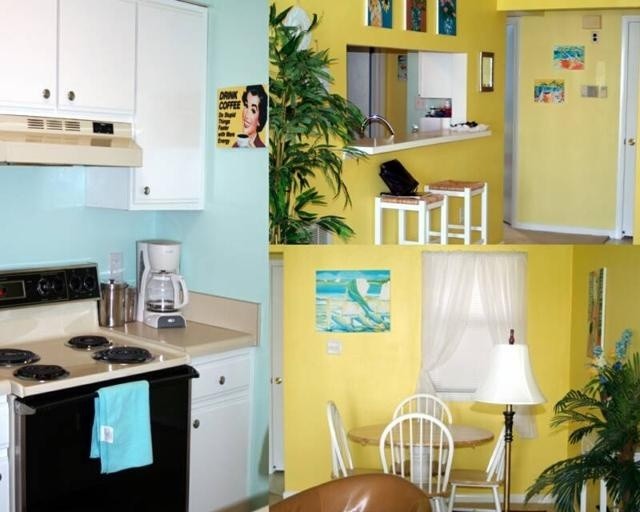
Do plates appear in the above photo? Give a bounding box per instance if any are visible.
[285,8,312,51]
[306,64,332,98]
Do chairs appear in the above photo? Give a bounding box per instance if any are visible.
[389,392,454,476]
[445,421,506,512]
[323,400,385,480]
[378,411,455,511]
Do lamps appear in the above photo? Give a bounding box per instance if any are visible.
[470,328,548,511]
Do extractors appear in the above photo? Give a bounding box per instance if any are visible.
[0,114,144,170]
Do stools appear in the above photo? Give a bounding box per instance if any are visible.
[424,179,489,244]
[372,192,449,244]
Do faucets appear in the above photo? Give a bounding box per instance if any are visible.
[359,115,395,137]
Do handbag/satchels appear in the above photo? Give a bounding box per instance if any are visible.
[380,160,419,196]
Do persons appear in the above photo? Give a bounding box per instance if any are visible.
[410,1,422,31]
[232,85,267,147]
[442,0,456,35]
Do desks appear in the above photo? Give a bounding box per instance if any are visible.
[347,418,494,484]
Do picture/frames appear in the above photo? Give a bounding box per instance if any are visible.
[479,51,495,92]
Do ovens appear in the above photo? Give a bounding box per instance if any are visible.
[15,367,198,512]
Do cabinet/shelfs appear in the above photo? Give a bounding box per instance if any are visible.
[186,339,254,510]
[0,2,134,130]
[83,1,209,212]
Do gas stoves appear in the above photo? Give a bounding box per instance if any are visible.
[0,329,184,397]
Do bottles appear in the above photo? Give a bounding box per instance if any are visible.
[101,279,127,327]
[429,105,437,117]
[411,124,420,134]
[443,100,452,114]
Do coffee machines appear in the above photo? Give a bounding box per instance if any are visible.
[133,237,190,331]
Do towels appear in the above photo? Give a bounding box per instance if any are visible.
[88,378,155,476]
